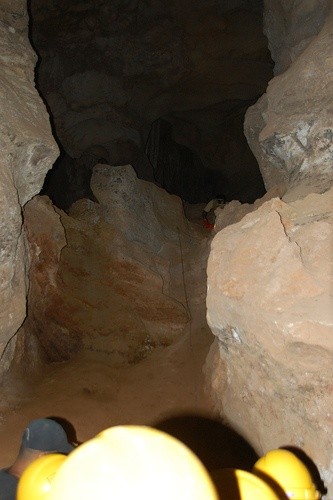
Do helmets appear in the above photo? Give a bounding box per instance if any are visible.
[253,448,321,500]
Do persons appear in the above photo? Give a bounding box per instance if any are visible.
[0,418,77,500]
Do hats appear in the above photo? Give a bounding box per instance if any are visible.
[15,425,281,500]
[22,419,78,456]
